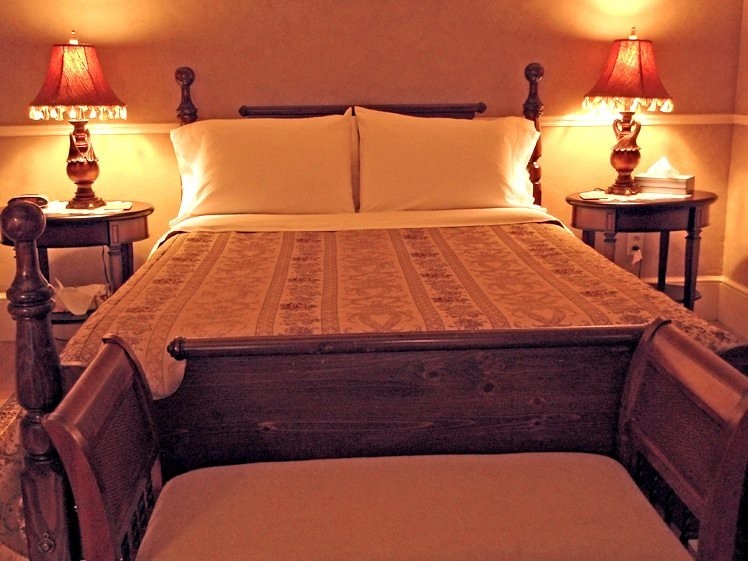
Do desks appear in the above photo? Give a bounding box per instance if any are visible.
[0,200,154,324]
[566,189,718,311]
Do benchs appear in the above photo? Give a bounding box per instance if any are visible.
[40,315,748,561]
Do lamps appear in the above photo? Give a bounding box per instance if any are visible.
[581,25,675,195]
[26,29,127,210]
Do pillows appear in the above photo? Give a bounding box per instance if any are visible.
[168,107,355,227]
[354,106,541,213]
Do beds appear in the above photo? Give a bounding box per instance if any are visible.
[1,62,748,561]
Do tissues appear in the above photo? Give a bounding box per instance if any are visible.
[633,155,695,195]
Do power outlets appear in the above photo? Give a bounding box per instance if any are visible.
[627,232,644,256]
